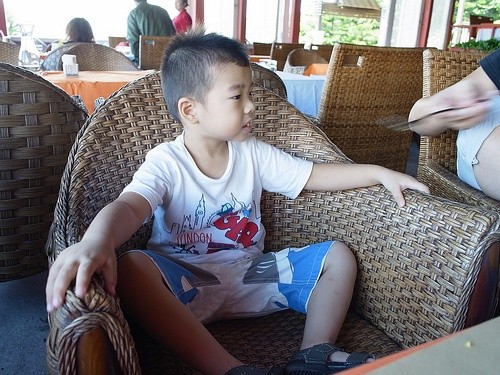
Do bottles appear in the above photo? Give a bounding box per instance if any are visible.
[16,23,44,76]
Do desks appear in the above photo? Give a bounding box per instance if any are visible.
[275,70,326,115]
[39,70,150,117]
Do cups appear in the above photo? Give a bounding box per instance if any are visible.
[259,59,277,73]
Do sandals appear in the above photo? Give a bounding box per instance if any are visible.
[223,365,268,375]
[284,343,377,375]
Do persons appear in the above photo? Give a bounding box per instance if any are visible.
[46,31,430,375]
[172,0,192,33]
[408,48,500,203]
[127,0,176,65]
[64,18,94,44]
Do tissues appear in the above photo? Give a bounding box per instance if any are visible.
[255,60,277,73]
[62,54,79,75]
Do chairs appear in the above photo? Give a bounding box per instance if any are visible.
[0,61,89,284]
[45,71,500,375]
[416,49,500,215]
[302,43,437,173]
[0,37,335,70]
